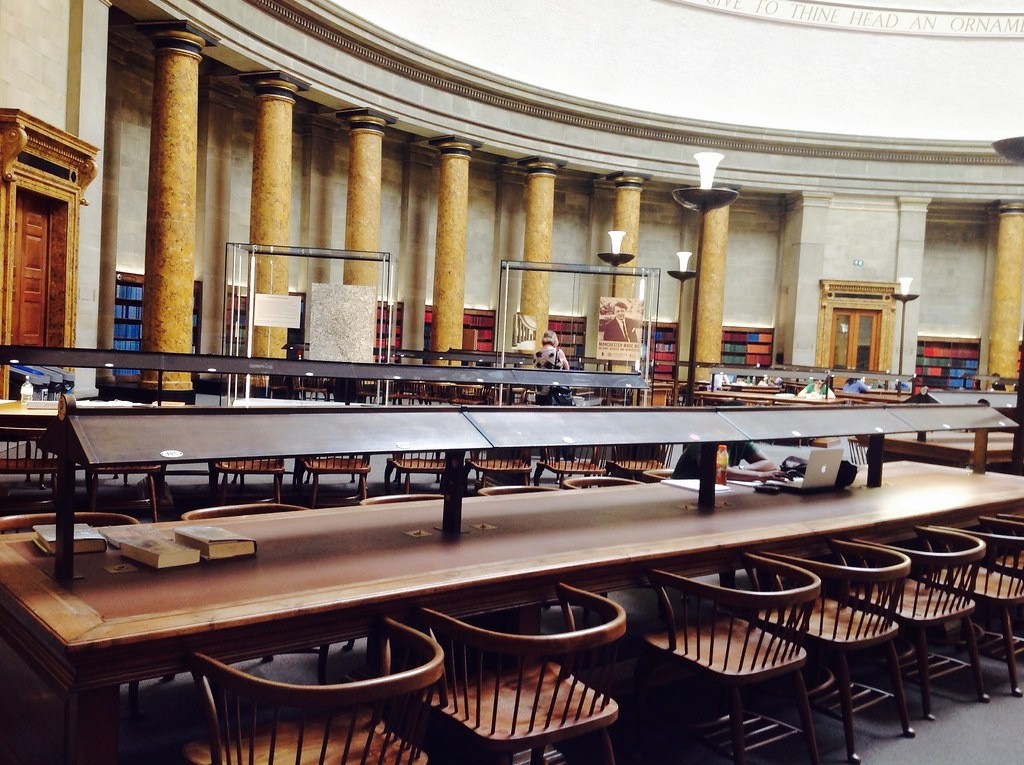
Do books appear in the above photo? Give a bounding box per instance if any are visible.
[721,332,773,365]
[375,308,402,360]
[113,285,142,376]
[120,538,201,568]
[99,524,174,548]
[464,314,494,352]
[915,346,979,395]
[174,526,258,560]
[31,524,107,556]
[548,321,584,366]
[651,332,676,378]
[424,310,432,350]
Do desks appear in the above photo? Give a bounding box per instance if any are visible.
[1,399,60,483]
[0,459,1024,765]
[642,376,910,405]
[855,431,1015,470]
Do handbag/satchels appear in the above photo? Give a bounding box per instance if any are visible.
[551,387,574,405]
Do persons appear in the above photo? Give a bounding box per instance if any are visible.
[533,330,576,460]
[991,373,1006,392]
[796,378,835,400]
[719,400,786,483]
[604,302,638,342]
[843,378,870,393]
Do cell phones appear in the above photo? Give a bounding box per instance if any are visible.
[755,485,780,494]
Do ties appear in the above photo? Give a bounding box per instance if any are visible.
[622,321,628,342]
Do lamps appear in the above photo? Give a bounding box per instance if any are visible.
[598,230,635,405]
[891,277,919,396]
[672,152,739,405]
[668,251,696,405]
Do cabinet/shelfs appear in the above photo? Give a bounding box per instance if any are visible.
[114,272,774,377]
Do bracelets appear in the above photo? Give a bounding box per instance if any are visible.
[822,395,824,399]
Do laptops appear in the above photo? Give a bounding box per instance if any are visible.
[765,447,844,489]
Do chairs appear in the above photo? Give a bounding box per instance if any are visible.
[976,513,1023,633]
[0,377,674,537]
[843,525,990,721]
[634,551,822,765]
[754,539,911,765]
[927,515,1024,697]
[183,615,444,765]
[412,582,627,765]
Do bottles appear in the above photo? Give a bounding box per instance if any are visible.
[751,376,756,385]
[747,375,750,385]
[20,376,34,405]
[716,444,728,486]
